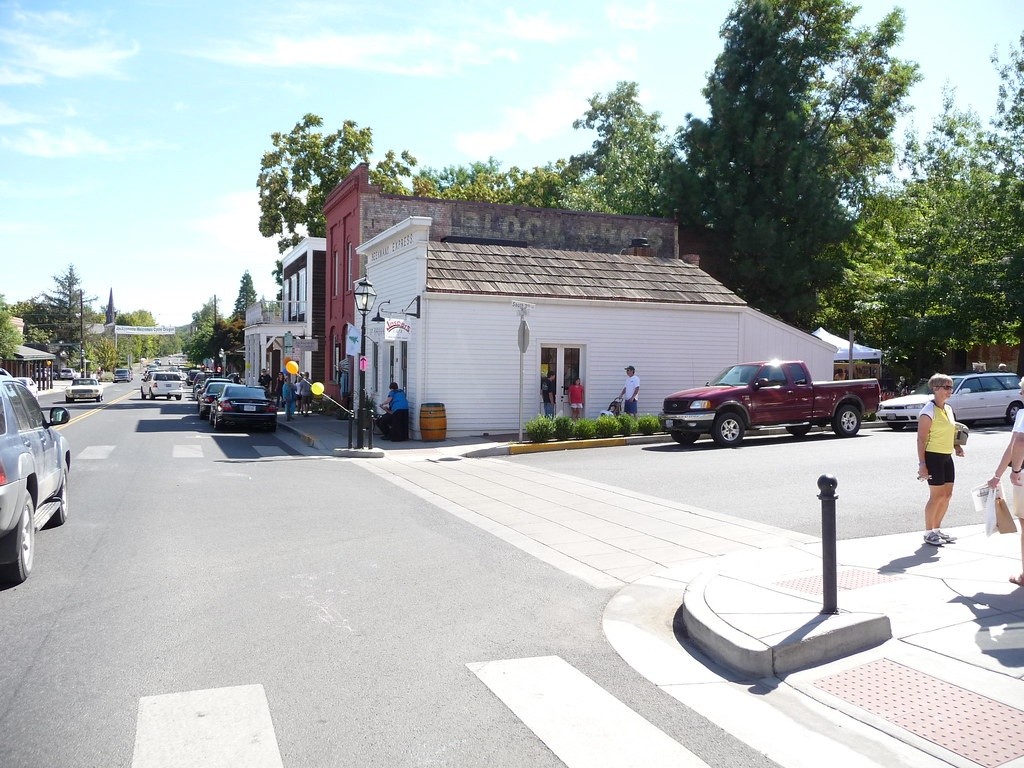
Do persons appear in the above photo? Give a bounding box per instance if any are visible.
[379,382,409,442]
[258,368,313,422]
[997,363,1008,384]
[569,378,584,419]
[375,395,392,440]
[894,376,910,397]
[618,365,640,418]
[834,368,846,380]
[541,370,556,420]
[988,376,1024,586]
[917,373,965,546]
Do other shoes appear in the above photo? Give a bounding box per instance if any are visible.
[380,436,390,440]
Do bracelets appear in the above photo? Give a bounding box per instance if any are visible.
[1011,467,1022,473]
[993,475,1000,479]
[918,461,926,466]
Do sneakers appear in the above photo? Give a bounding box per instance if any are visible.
[923,530,958,547]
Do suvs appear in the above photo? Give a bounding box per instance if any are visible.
[1,367,71,583]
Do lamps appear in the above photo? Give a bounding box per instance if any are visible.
[370,300,390,323]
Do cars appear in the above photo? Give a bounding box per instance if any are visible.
[65,378,104,402]
[209,384,278,432]
[140,359,188,400]
[36,367,81,381]
[13,376,39,401]
[875,373,1024,431]
[187,369,250,420]
[113,369,132,382]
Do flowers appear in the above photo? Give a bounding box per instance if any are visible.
[363,384,377,417]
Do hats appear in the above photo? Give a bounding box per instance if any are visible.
[625,366,635,370]
[389,383,397,389]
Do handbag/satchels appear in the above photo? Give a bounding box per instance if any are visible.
[954,422,969,445]
[995,497,1017,534]
[985,487,998,538]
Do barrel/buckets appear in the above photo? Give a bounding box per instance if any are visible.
[420,403,447,441]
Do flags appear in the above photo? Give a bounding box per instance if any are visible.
[345,322,362,356]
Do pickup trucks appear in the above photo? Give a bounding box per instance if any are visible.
[658,359,884,447]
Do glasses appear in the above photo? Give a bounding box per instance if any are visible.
[627,370,629,371]
[931,386,953,390]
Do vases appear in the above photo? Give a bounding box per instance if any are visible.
[361,410,374,429]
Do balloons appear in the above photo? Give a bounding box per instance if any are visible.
[285,360,299,375]
[311,381,325,396]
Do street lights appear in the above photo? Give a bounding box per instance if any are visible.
[353,274,379,449]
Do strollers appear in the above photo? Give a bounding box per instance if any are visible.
[596,395,625,419]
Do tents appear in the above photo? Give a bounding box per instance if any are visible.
[811,326,882,377]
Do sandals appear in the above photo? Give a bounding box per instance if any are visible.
[1009,574,1024,586]
